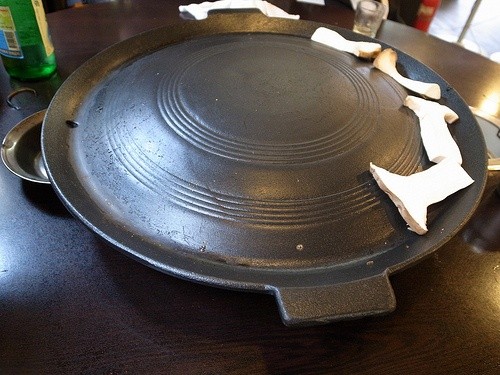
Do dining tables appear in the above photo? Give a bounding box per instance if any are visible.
[0,0,500,375]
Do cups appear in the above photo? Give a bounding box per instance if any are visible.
[352,0,384,38]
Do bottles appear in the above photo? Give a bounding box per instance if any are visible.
[0,0,57,79]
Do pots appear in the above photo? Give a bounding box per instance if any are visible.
[39,15,490,327]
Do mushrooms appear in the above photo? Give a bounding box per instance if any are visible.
[310,26,480,235]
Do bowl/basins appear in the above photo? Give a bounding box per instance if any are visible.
[1,109,53,184]
